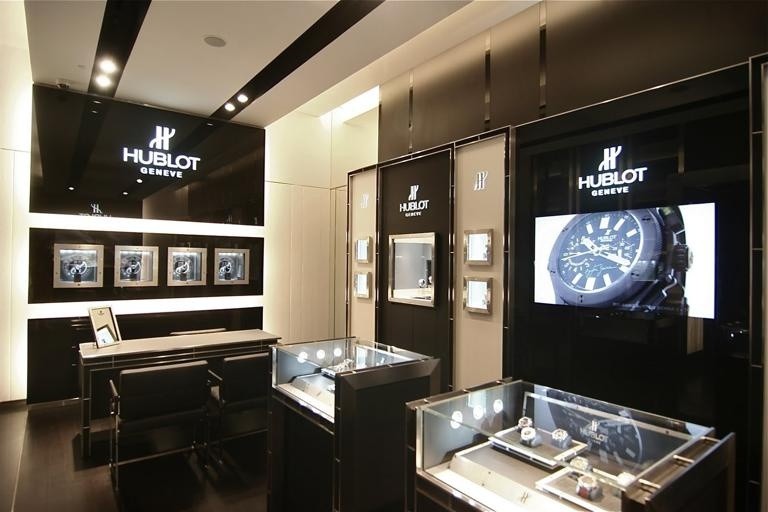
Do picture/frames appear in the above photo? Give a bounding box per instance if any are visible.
[214,248,249,284]
[97,324,117,341]
[167,247,207,286]
[53,244,104,288]
[114,246,159,287]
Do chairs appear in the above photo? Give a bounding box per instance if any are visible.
[197,353,269,500]
[109,361,208,512]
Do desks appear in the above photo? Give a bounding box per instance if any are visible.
[79,329,282,469]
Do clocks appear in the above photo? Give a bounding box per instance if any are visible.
[547,388,692,473]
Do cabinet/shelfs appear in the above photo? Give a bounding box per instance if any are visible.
[405,375,735,512]
[268,336,440,512]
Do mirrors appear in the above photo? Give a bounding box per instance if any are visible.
[462,276,493,314]
[88,305,122,349]
[354,272,371,299]
[463,229,493,266]
[388,232,436,307]
[354,236,372,263]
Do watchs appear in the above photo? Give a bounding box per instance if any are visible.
[570,458,589,476]
[520,428,537,446]
[551,429,571,447]
[577,475,599,499]
[518,417,532,430]
